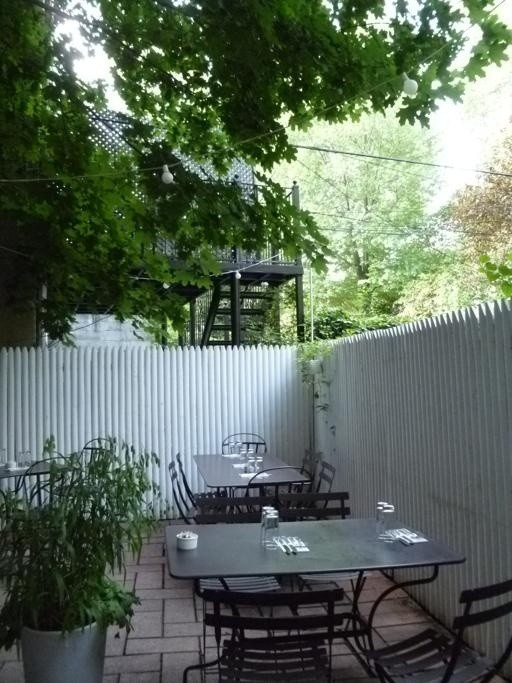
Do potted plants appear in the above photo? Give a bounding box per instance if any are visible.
[0,431,166,682]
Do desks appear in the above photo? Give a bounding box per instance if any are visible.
[169,517,466,683]
[0,459,77,512]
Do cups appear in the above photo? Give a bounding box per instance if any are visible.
[229,439,264,473]
[381,504,397,542]
[375,500,389,535]
[261,505,279,550]
[176,532,198,550]
[1,448,35,472]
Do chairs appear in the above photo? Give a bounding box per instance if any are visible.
[169,433,372,623]
[200,588,344,683]
[364,579,511,683]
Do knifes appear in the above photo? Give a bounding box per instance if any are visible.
[277,536,297,554]
[390,529,414,546]
